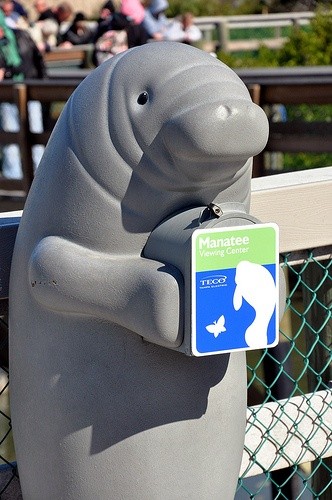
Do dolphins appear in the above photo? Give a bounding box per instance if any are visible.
[8,39,287,500]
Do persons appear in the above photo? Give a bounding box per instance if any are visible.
[0,0,204,84]
